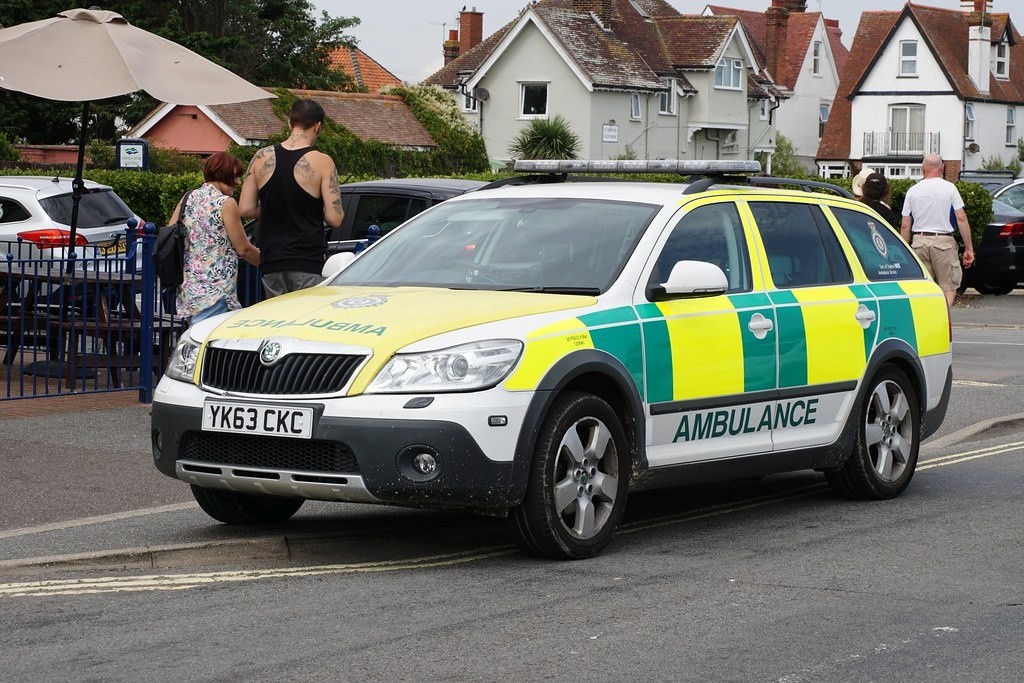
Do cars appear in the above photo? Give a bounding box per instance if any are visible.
[957,199,1024,297]
[236,178,492,306]
[1,176,146,318]
[992,178,1024,215]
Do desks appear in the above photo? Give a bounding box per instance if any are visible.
[0,266,143,390]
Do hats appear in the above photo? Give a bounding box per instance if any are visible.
[865,173,887,189]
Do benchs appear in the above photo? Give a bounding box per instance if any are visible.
[0,310,187,390]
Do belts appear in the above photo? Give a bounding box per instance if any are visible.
[915,232,954,236]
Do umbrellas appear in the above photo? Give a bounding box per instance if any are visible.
[0,5,279,271]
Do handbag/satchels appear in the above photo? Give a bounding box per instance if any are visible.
[152,190,192,285]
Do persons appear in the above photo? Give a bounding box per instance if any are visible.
[851,168,892,223]
[239,98,345,300]
[901,154,974,309]
[169,151,260,326]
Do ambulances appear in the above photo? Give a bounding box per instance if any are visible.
[146,156,956,557]
